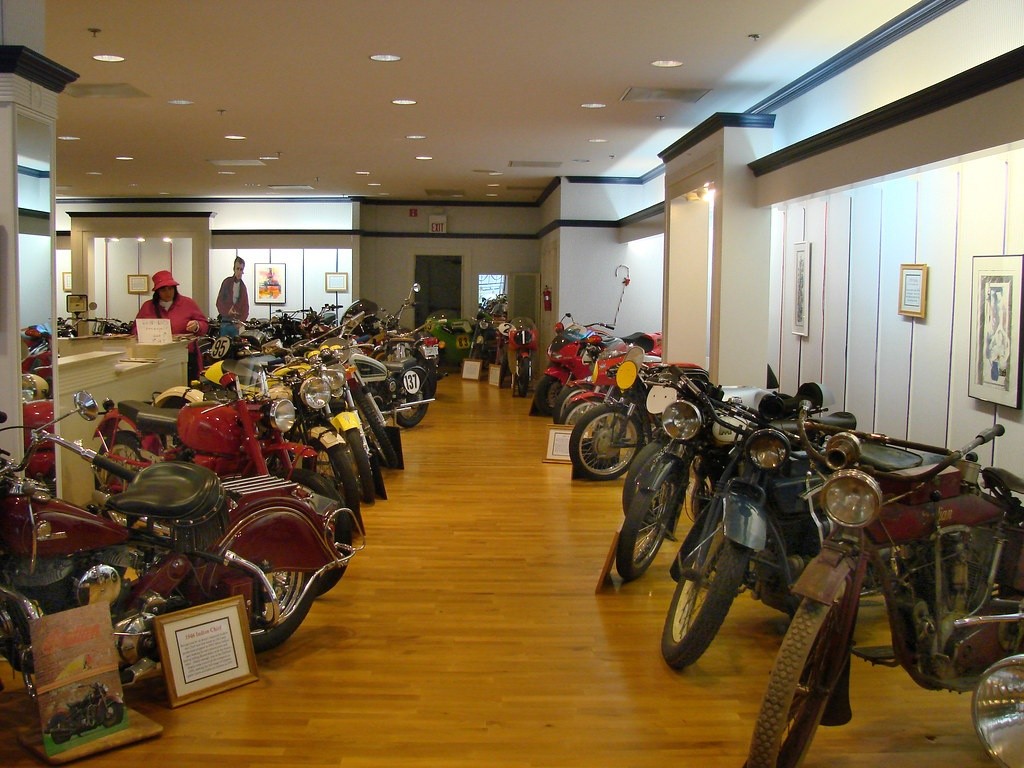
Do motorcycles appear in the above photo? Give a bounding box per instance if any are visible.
[0,281,1024,768]
[44,680,125,744]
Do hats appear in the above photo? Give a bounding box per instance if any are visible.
[151,270,180,291]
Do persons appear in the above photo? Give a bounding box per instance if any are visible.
[216,256,250,337]
[133,271,210,388]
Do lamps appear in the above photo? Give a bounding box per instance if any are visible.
[697,188,711,200]
[708,181,715,193]
[685,193,700,203]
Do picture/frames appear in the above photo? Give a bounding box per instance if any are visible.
[254,262,286,304]
[791,241,810,337]
[127,274,149,294]
[541,424,592,464]
[461,358,482,382]
[897,264,928,319]
[967,254,1024,409]
[153,594,259,708]
[488,364,502,388]
[62,272,72,291]
[324,272,349,293]
